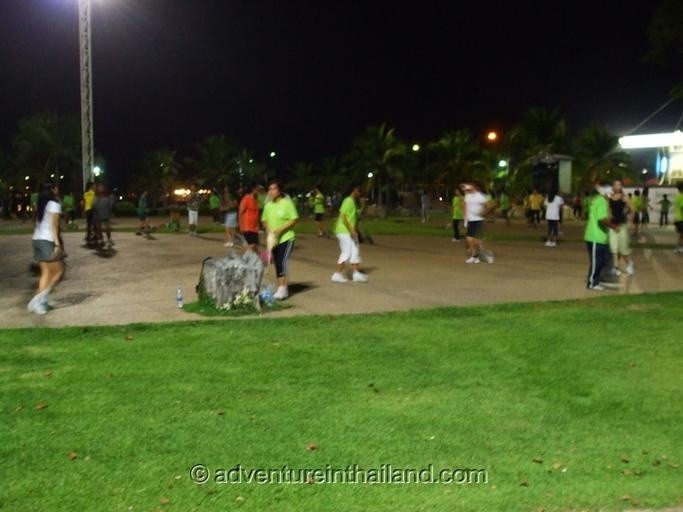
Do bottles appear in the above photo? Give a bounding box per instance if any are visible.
[258,283,274,306]
[176,288,184,309]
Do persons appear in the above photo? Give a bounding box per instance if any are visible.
[135,190,151,235]
[420,178,683,291]
[28,179,114,314]
[187,182,367,300]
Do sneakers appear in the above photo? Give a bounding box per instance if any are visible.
[352,271,369,282]
[222,241,233,247]
[464,256,481,264]
[272,285,289,301]
[26,294,51,316]
[543,240,556,248]
[585,259,636,291]
[330,271,349,283]
[484,249,494,265]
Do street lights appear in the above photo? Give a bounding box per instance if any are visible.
[485,132,499,194]
[413,144,428,197]
[368,172,376,203]
